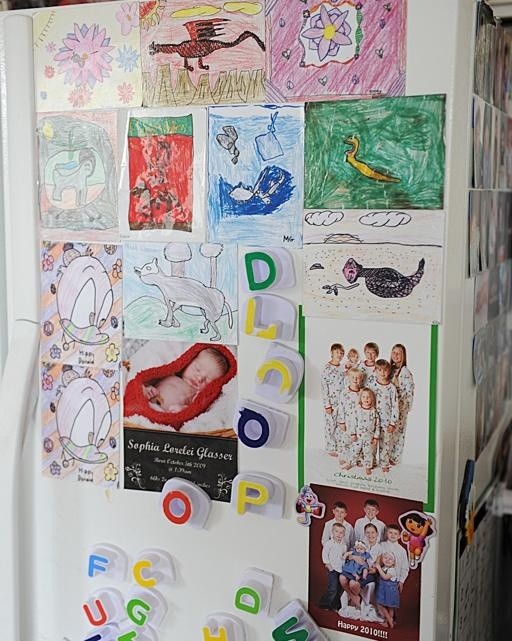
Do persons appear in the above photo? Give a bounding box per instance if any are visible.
[142,348,230,414]
[319,499,409,629]
[320,342,415,475]
